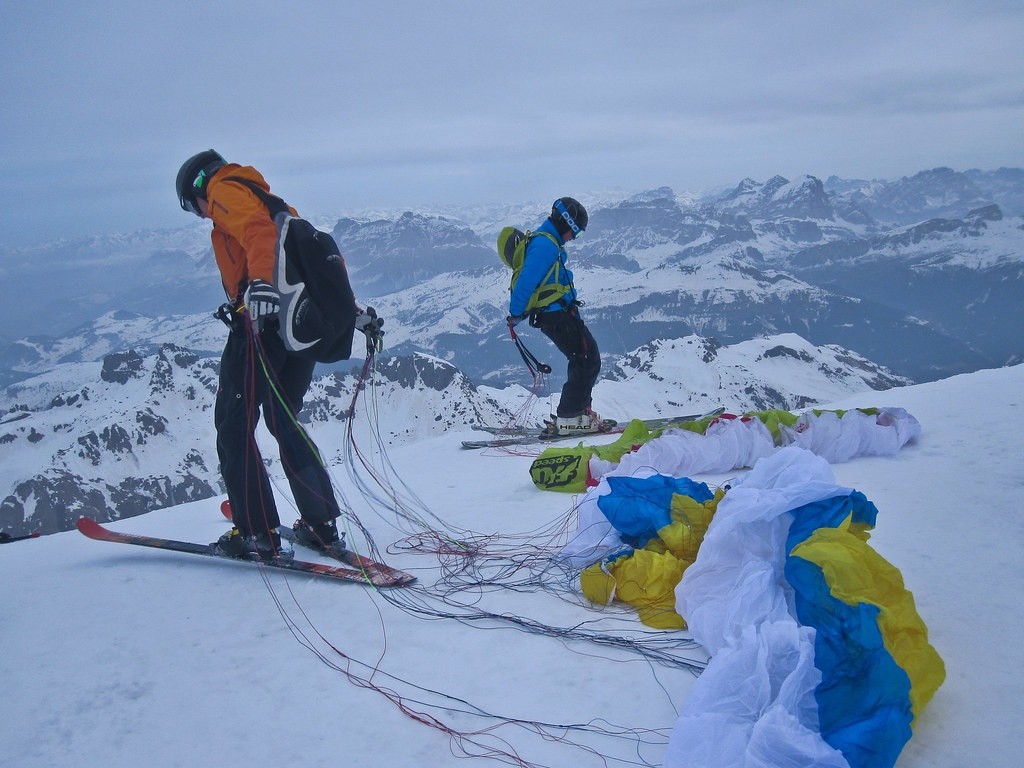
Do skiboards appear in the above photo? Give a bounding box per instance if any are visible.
[77,496,416,587]
[462,405,728,450]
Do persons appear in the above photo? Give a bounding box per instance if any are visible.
[504,196,616,439]
[178,150,346,562]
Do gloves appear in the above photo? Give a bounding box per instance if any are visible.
[507,314,528,327]
[354,306,385,337]
[244,279,282,335]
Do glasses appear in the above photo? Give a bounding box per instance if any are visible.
[572,226,586,239]
[182,194,202,217]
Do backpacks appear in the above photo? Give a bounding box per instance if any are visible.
[220,177,356,363]
[497,227,571,318]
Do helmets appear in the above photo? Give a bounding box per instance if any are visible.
[175,149,228,196]
[551,197,588,235]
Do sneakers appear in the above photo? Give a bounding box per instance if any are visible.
[556,408,616,435]
[292,516,339,545]
[218,527,281,555]
